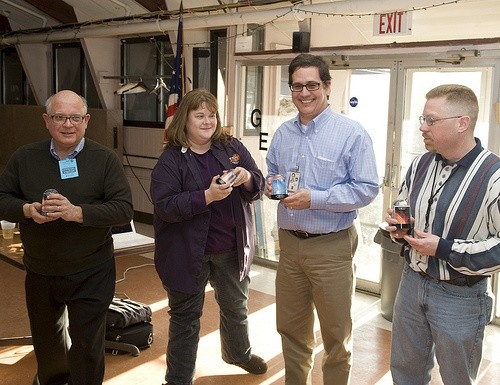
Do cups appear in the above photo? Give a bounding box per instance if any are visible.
[1,221,16,239]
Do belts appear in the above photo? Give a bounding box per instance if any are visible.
[418,270,466,287]
[288,230,334,239]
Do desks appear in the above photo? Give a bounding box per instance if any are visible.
[0,213,155,357]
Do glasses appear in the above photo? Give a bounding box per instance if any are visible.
[289,80,325,92]
[419,116,462,126]
[51,114,87,126]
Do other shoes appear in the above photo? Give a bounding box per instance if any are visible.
[230,354,267,374]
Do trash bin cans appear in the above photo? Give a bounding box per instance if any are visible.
[379,222,410,322]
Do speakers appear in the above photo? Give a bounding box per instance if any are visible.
[292,32,309,52]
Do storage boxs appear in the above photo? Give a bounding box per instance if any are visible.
[0,345,38,385]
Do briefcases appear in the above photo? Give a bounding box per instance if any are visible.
[105,322,153,356]
[106,296,152,328]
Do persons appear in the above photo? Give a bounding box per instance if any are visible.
[0,89,134,385]
[264,53,379,385]
[385,84,500,385]
[148,88,267,385]
